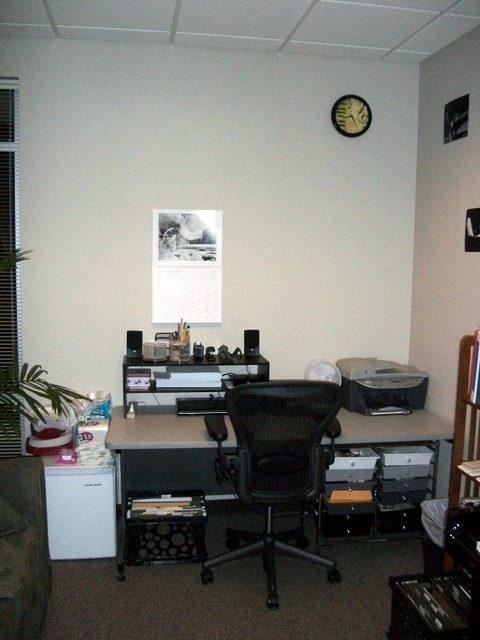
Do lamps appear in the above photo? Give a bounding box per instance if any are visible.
[200,379,345,609]
[0,458,52,640]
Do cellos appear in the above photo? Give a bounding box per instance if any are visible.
[336,357,429,416]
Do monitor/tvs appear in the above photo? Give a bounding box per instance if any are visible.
[462,460,479,472]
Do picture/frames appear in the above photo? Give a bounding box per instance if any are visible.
[106,404,455,582]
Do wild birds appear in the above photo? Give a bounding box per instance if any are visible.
[176,398,228,415]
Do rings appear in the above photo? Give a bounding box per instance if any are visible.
[173,317,192,342]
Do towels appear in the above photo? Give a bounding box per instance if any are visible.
[420,498,480,572]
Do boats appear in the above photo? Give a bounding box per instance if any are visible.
[244,330,260,356]
[127,331,143,359]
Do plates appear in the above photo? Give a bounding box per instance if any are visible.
[421,334,480,573]
[38,456,118,561]
[122,356,271,416]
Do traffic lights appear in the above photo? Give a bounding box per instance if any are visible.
[0,499,28,537]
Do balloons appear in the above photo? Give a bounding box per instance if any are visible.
[331,95,373,138]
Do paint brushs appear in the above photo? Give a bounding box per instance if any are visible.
[126,402,137,420]
